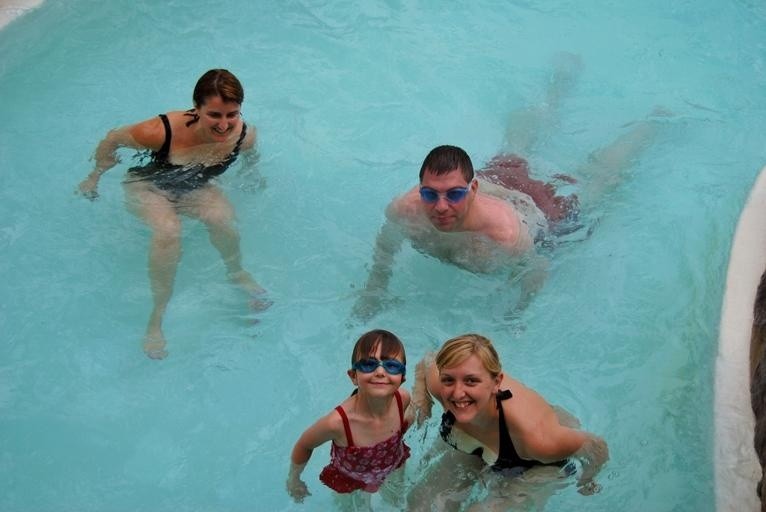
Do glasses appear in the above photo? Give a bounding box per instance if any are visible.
[352,357,406,375]
[419,177,475,204]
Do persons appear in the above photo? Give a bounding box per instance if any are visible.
[285,328,417,512]
[342,52,676,331]
[78,69,273,360]
[409,334,608,511]
